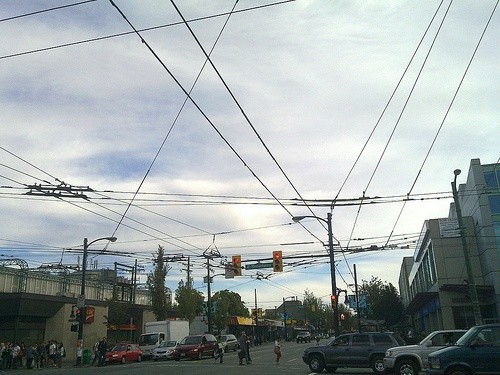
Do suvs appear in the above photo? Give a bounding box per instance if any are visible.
[176,334,219,360]
[421,324,500,375]
[382,330,470,375]
[214,335,239,353]
[301,332,407,375]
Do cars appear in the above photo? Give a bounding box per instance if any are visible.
[150,340,182,361]
[101,343,144,364]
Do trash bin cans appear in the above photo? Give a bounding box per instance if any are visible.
[83,349,90,364]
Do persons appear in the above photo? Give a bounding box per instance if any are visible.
[443,335,450,345]
[253,334,261,346]
[239,331,252,365]
[0,339,66,371]
[91,337,108,367]
[274,337,282,365]
[215,336,225,364]
[316,334,320,345]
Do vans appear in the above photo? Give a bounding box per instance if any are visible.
[296,332,311,343]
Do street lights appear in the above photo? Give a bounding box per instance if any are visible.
[283,296,295,342]
[450,168,482,325]
[72,236,117,366]
[292,213,340,340]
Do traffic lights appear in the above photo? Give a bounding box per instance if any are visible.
[76,309,81,321]
[273,251,283,272]
[331,296,336,310]
[73,325,79,332]
[232,256,241,276]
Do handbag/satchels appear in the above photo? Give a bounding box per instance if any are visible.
[274,347,279,354]
[238,348,247,359]
[31,359,35,367]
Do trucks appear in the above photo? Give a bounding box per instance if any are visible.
[138,320,189,360]
